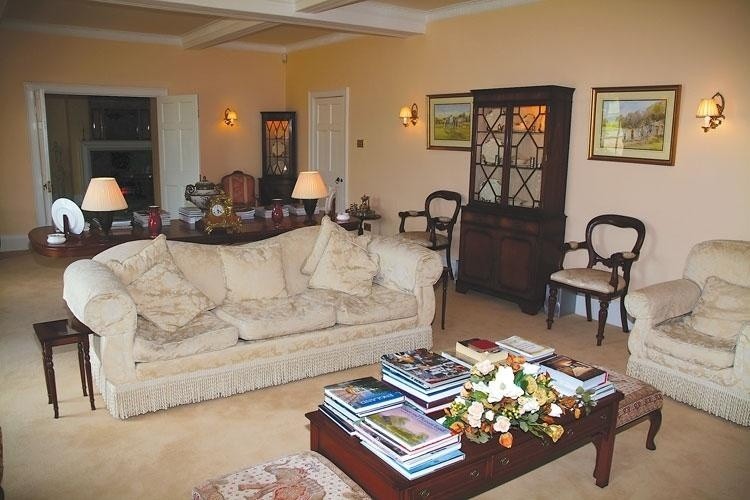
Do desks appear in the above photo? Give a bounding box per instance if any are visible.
[26,208,363,264]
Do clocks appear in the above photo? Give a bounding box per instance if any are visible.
[200,187,244,236]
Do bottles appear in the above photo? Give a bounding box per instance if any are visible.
[47,234,66,244]
[337,212,351,221]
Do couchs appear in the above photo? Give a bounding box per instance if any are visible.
[58,222,448,426]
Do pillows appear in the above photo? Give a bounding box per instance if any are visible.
[679,274,750,343]
[102,232,188,318]
[124,260,216,335]
[213,241,292,308]
[306,229,382,299]
[298,212,373,276]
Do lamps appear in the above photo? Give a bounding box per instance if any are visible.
[398,101,420,129]
[289,168,331,227]
[694,91,729,134]
[80,175,130,243]
[222,107,239,130]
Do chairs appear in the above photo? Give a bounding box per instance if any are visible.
[545,212,647,348]
[391,188,463,282]
[219,169,266,215]
[623,236,750,434]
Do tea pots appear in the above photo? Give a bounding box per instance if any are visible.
[271,198,284,227]
[147,206,163,239]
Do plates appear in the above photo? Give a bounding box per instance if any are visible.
[51,198,84,235]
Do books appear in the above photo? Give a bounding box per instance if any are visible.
[319,332,616,479]
[109,204,319,226]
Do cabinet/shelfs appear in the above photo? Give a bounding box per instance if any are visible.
[257,110,302,208]
[454,84,577,318]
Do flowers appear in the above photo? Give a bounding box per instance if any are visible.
[432,341,602,457]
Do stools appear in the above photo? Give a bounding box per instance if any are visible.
[585,360,663,480]
[29,316,98,421]
[187,449,374,500]
[426,263,453,332]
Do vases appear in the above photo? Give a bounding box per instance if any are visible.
[147,203,164,241]
[272,199,285,225]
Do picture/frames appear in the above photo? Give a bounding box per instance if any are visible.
[586,81,684,167]
[423,91,476,154]
